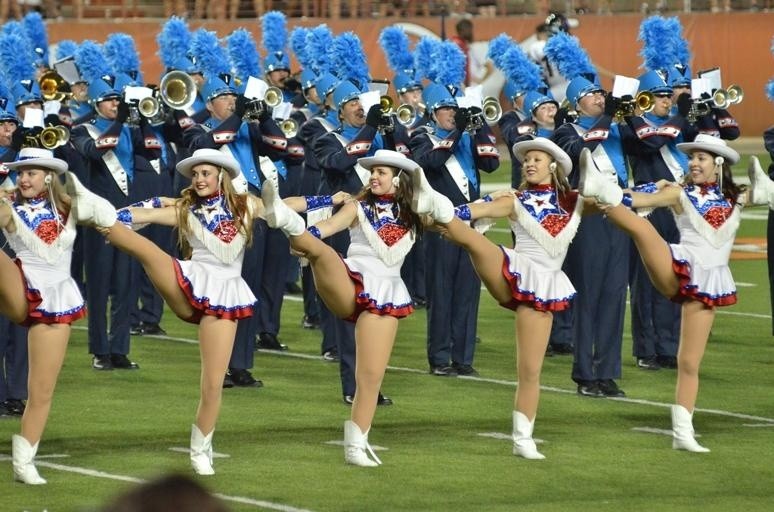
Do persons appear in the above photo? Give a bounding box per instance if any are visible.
[410,136,675,461]
[575,135,765,456]
[762,126,773,337]
[747,156,774,210]
[531,22,570,108]
[260,148,517,471]
[0,51,694,413]
[63,146,351,479]
[0,146,188,486]
[447,18,494,88]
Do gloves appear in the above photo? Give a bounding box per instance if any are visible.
[452,109,473,132]
[363,105,385,128]
[703,91,723,114]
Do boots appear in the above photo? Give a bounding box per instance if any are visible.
[577,145,626,209]
[746,158,774,207]
[189,424,218,479]
[261,178,305,241]
[343,420,379,469]
[669,406,711,456]
[511,409,547,460]
[410,166,456,228]
[9,432,46,486]
[62,171,119,238]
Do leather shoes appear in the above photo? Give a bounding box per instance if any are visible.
[254,334,289,351]
[545,343,574,355]
[92,353,138,370]
[577,379,626,397]
[302,315,321,328]
[324,349,340,361]
[130,322,167,336]
[223,369,263,388]
[637,356,679,369]
[343,393,392,405]
[409,290,430,310]
[432,363,479,377]
[0,393,26,417]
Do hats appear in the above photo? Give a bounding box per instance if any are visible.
[0,70,145,121]
[0,147,70,175]
[169,58,362,111]
[357,149,421,177]
[674,132,743,162]
[176,147,243,180]
[511,136,573,179]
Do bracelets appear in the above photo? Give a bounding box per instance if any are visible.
[304,196,333,213]
[307,226,321,239]
[631,182,656,194]
[117,210,132,222]
[455,205,472,221]
[622,193,632,207]
[482,195,492,202]
[132,198,162,208]
